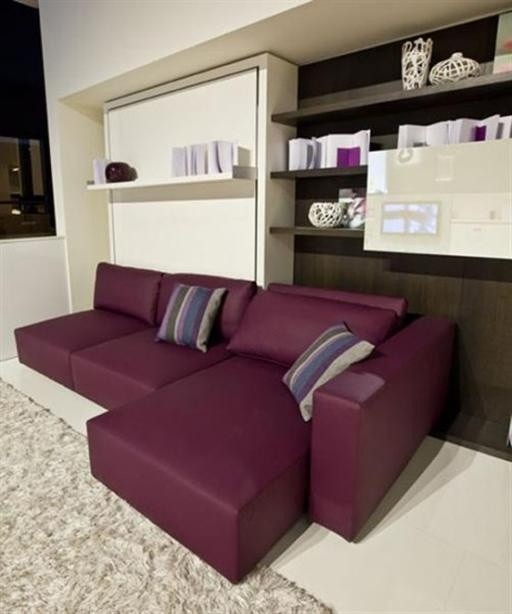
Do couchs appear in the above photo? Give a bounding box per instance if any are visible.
[14,260,456,587]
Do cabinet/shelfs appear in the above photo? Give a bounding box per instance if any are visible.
[268,1,512,240]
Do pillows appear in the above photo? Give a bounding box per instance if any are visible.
[152,282,228,353]
[281,321,377,426]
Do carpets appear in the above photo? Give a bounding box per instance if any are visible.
[0,375,333,613]
